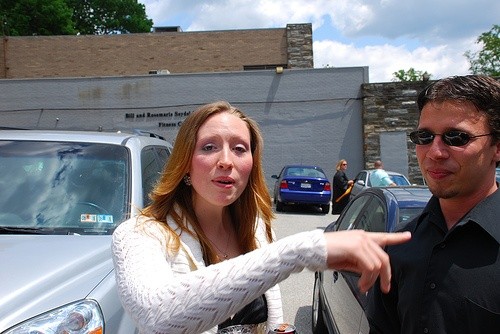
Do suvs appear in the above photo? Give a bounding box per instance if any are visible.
[0,125,173,334]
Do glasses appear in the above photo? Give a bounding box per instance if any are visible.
[406,127,489,147]
[342,164,346,166]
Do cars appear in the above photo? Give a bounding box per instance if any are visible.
[312,186,433,334]
[347,169,418,203]
[270,164,332,214]
[495,167,500,187]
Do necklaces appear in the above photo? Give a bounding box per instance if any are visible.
[210,241,231,257]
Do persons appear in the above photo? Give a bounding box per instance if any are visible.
[376,75,500,334]
[332,160,353,214]
[370,161,397,188]
[111,101,411,332]
